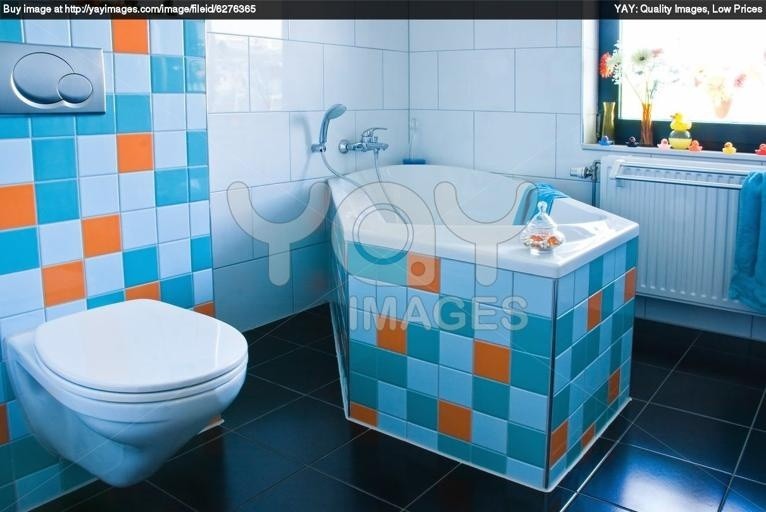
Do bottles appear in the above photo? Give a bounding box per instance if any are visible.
[520,201,564,255]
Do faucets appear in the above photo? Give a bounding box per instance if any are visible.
[338,125,391,154]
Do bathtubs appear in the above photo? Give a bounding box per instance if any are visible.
[327,166,640,492]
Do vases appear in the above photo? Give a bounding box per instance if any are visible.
[640,103,653,148]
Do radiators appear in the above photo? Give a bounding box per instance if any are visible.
[570,155,766,316]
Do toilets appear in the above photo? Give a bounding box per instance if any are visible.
[3,288,248,488]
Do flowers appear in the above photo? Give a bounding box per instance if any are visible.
[598,38,670,142]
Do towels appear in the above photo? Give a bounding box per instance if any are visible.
[727,170,765,317]
[513,183,571,226]
